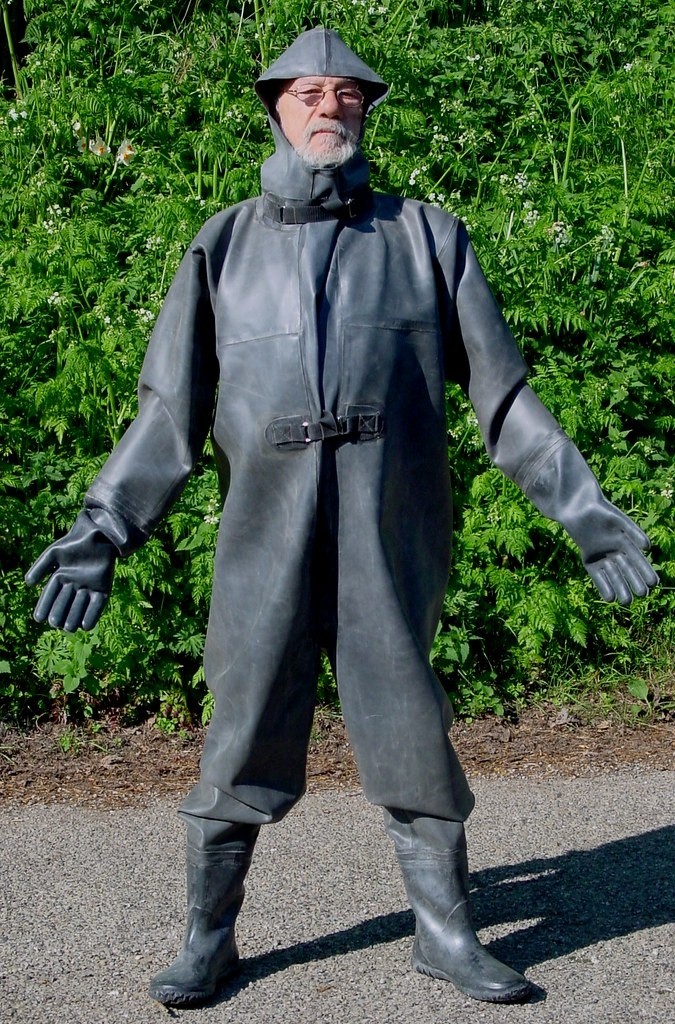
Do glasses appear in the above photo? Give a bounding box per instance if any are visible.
[281,83,364,108]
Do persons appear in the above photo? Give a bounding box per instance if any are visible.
[20,19,659,1008]
